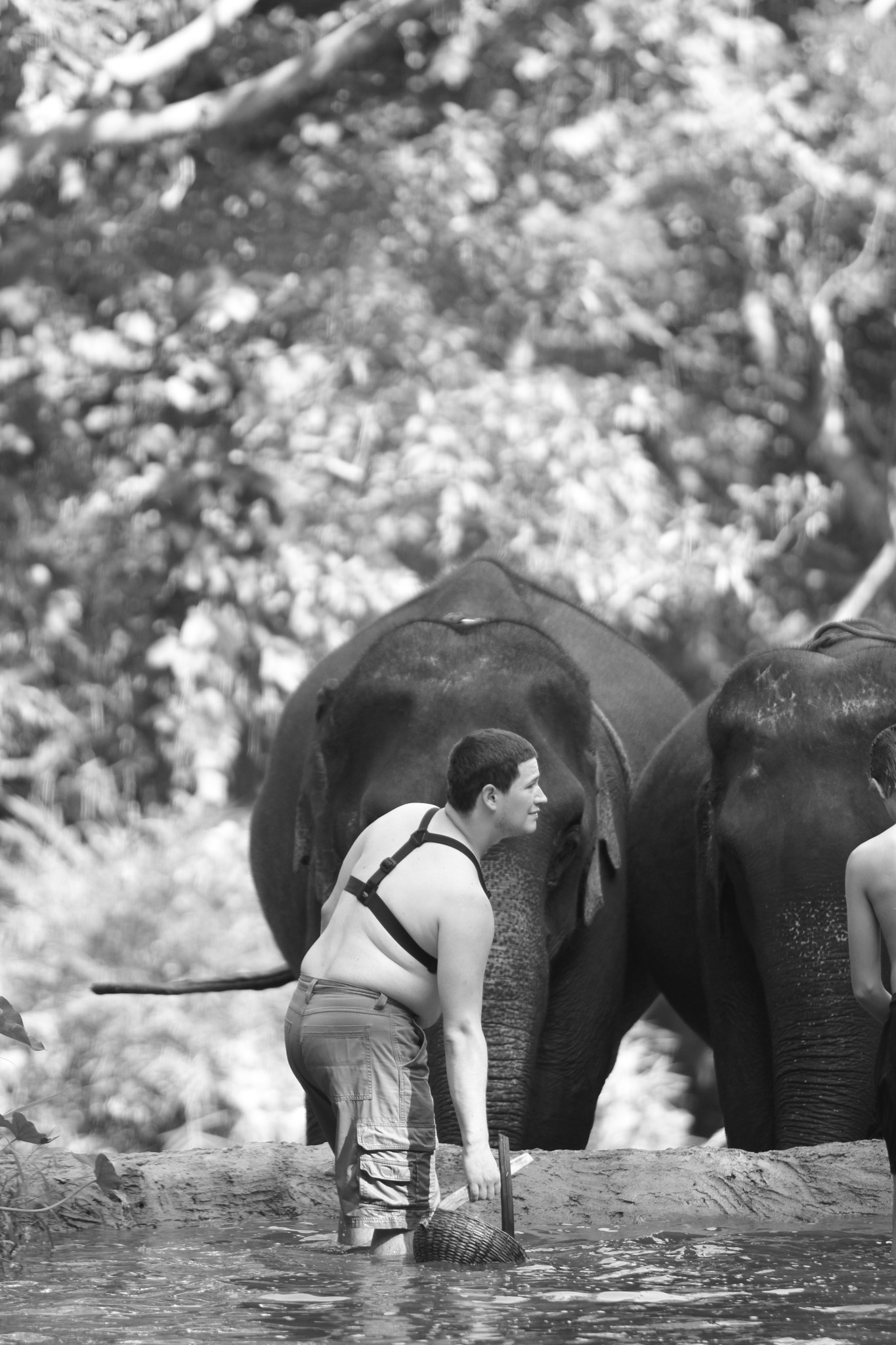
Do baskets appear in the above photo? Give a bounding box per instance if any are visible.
[412,1209,528,1267]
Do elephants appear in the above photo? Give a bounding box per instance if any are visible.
[247,555,895,1174]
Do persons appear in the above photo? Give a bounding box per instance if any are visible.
[282,727,550,1259]
[844,723,896,1263]
